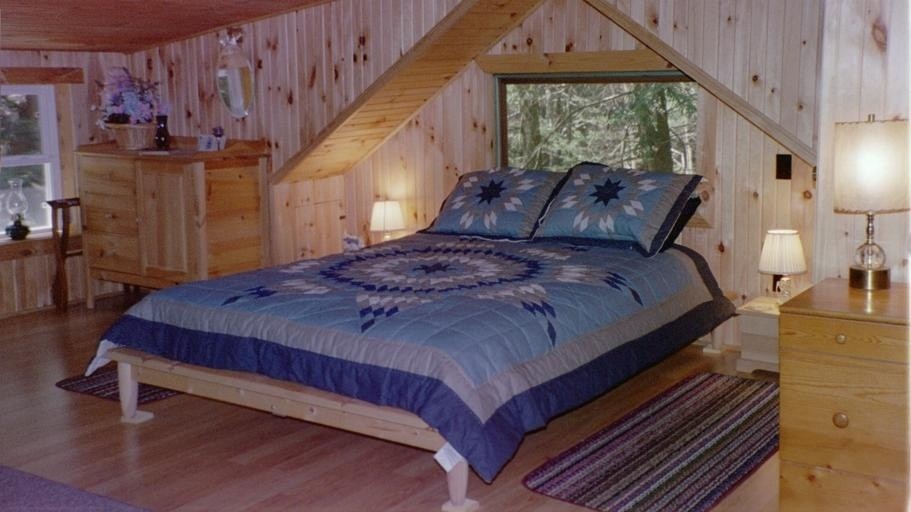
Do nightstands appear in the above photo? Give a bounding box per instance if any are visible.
[777,269,910,511]
[735,297,795,374]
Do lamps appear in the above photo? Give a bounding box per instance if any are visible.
[756,228,807,298]
[830,111,909,291]
[368,200,407,242]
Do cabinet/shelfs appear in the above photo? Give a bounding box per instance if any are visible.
[67,135,273,313]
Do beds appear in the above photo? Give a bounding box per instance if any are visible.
[81,164,728,512]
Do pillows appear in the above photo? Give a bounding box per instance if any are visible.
[661,196,700,254]
[418,170,571,244]
[532,160,703,260]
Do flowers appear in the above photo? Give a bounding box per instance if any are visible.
[87,65,174,125]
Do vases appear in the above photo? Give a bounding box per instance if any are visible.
[105,124,159,151]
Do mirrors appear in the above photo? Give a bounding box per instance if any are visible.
[215,26,255,118]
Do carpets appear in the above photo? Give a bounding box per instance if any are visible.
[1,466,150,511]
[54,366,182,405]
[523,367,780,512]
